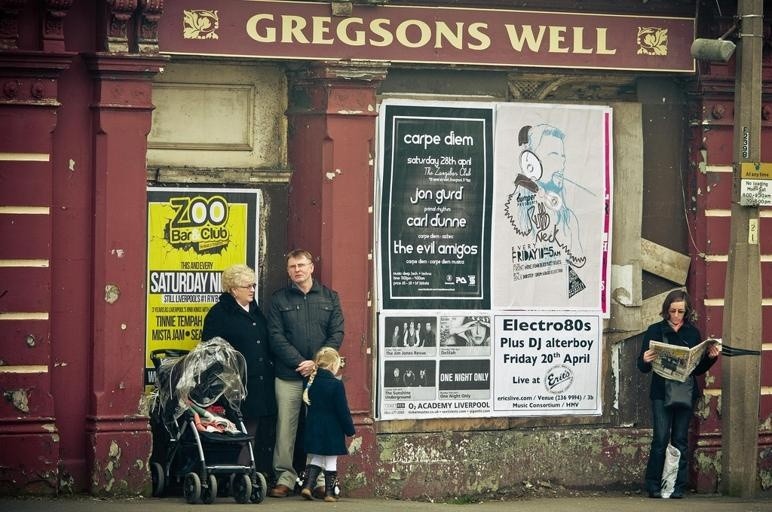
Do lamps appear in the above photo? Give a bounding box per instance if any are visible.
[690,23,744,62]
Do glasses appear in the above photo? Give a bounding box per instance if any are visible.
[290,263,312,268]
[239,284,256,289]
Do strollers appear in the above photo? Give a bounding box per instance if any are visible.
[149,346,268,505]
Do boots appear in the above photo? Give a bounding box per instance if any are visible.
[301,464,340,502]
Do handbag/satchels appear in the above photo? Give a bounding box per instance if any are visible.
[663,377,694,409]
[659,443,681,499]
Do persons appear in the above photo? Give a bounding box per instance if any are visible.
[197,395,242,433]
[298,345,358,502]
[635,289,723,500]
[200,263,277,496]
[265,245,346,500]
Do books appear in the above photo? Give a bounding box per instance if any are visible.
[648,337,722,383]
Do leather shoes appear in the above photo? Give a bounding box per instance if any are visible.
[650,491,682,498]
[270,485,289,497]
